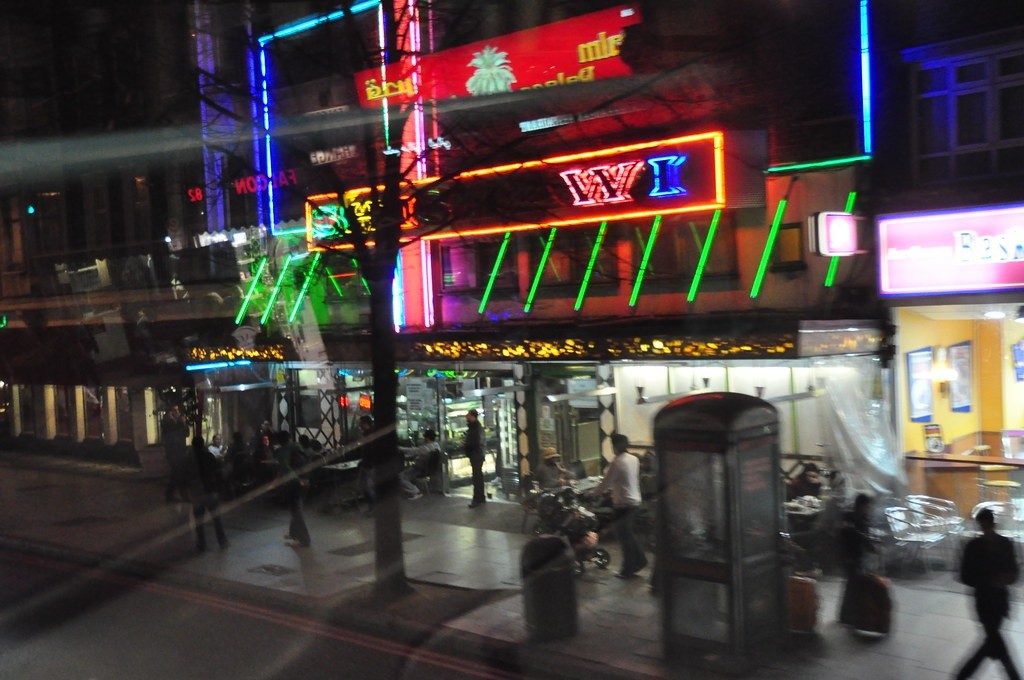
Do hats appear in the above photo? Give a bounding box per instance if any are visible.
[359,415,374,423]
[829,471,844,482]
[804,463,818,472]
[541,447,561,460]
[852,493,872,510]
[422,429,439,437]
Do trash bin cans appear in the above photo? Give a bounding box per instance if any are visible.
[517,534,578,644]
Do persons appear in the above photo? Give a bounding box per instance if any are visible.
[839,493,878,578]
[952,509,1023,680]
[182,415,440,553]
[787,462,820,500]
[538,446,576,489]
[594,434,647,578]
[460,409,488,507]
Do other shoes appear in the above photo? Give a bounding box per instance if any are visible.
[196,546,206,553]
[287,541,311,548]
[219,541,231,550]
[614,570,633,579]
[634,560,648,573]
[407,492,423,501]
[165,497,179,503]
[468,501,480,508]
[182,496,191,502]
[284,534,293,539]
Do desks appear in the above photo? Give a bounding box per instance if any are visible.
[536,478,603,494]
[324,461,363,510]
[787,499,854,518]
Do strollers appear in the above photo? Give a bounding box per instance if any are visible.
[531,488,611,574]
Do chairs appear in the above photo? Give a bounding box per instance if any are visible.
[219,450,450,502]
[838,494,1024,574]
[521,470,542,531]
